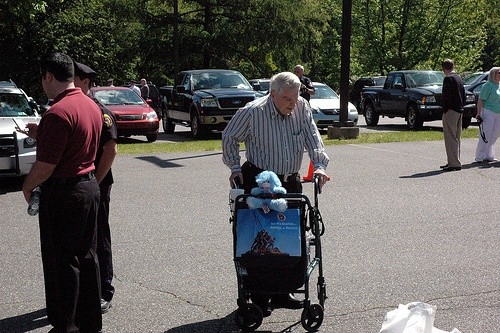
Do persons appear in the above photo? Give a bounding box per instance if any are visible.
[292,65,316,102]
[222,71,330,317]
[474,67,500,164]
[139,78,149,99]
[439,59,466,171]
[21,51,118,333]
[107,77,114,87]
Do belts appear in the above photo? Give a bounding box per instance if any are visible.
[245,160,300,178]
[45,171,93,182]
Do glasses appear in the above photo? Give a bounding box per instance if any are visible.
[37,71,53,84]
[495,72,500,74]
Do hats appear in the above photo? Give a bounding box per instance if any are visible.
[72,58,97,81]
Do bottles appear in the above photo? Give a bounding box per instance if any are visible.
[28,187,40,216]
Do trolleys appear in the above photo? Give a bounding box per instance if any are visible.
[227,175,329,333]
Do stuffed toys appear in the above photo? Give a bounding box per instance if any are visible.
[247,170,288,213]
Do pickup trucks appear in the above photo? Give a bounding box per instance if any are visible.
[157,69,264,141]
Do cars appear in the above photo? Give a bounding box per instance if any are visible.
[349,70,491,131]
[0,79,55,179]
[249,79,270,96]
[309,82,358,127]
[87,86,160,142]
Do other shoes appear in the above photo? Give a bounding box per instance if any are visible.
[440,164,448,168]
[100,297,110,313]
[477,159,495,168]
[491,159,500,167]
[443,166,461,172]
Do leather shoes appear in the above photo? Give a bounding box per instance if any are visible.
[252,301,272,316]
[273,293,304,309]
